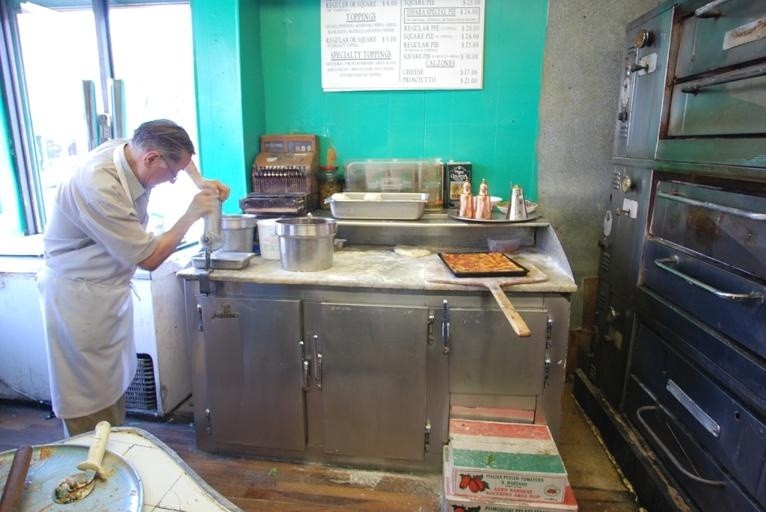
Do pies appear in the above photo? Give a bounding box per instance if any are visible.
[52,473,94,504]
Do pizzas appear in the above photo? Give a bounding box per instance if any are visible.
[441,251,524,273]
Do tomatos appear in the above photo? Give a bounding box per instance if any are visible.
[460,475,485,493]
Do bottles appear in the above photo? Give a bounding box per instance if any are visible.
[460,178,492,220]
[506,184,527,220]
[319,164,343,208]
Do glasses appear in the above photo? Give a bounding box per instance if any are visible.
[159,154,177,184]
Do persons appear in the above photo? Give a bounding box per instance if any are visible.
[36,119,230,440]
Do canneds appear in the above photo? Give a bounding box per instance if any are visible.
[317,165,341,210]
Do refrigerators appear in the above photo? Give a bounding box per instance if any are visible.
[0,223,197,422]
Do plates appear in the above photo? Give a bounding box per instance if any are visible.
[498,200,538,214]
[1,444,142,512]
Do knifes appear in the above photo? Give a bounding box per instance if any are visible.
[76,421,111,486]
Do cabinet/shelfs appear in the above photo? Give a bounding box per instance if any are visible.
[184,281,438,483]
[442,296,570,401]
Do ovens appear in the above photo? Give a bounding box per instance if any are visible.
[583,0,763,496]
